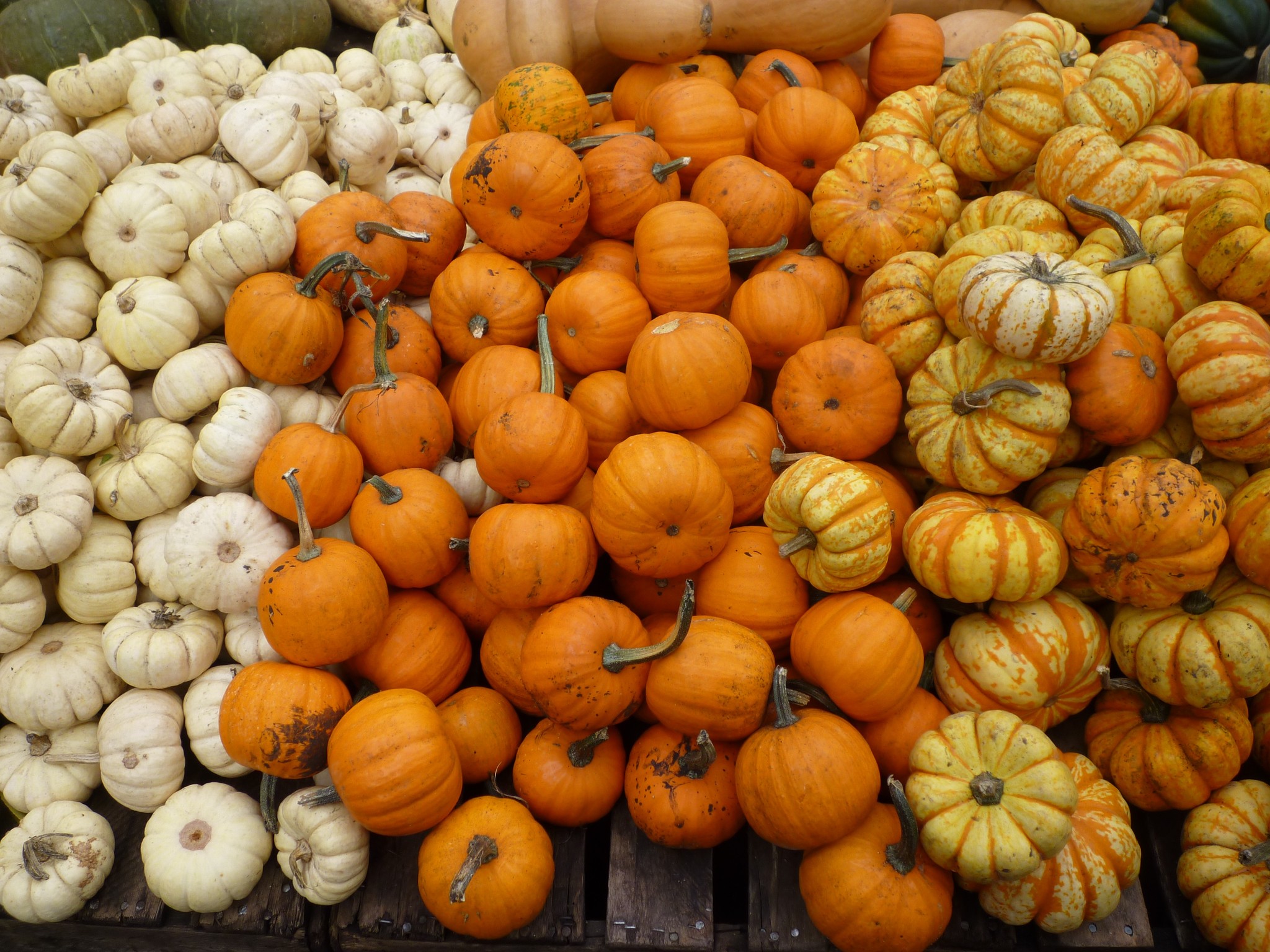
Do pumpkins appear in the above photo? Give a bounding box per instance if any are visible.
[0,0,1270,952]
